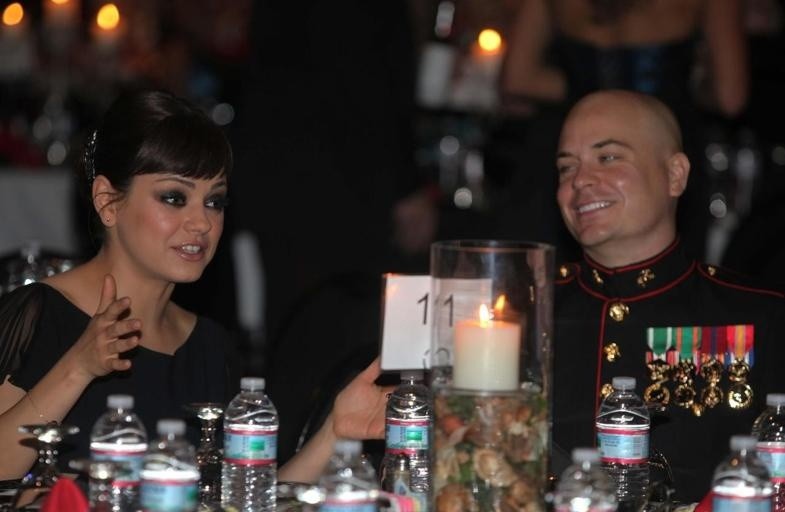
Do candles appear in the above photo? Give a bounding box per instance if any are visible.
[451,294,525,394]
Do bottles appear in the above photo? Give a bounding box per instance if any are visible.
[317,372,433,512]
[87,375,278,512]
[553,378,784,512]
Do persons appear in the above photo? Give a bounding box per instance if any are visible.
[0,91,399,486]
[0,0,785,386]
[491,86,785,512]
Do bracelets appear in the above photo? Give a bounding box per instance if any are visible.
[27,391,51,424]
